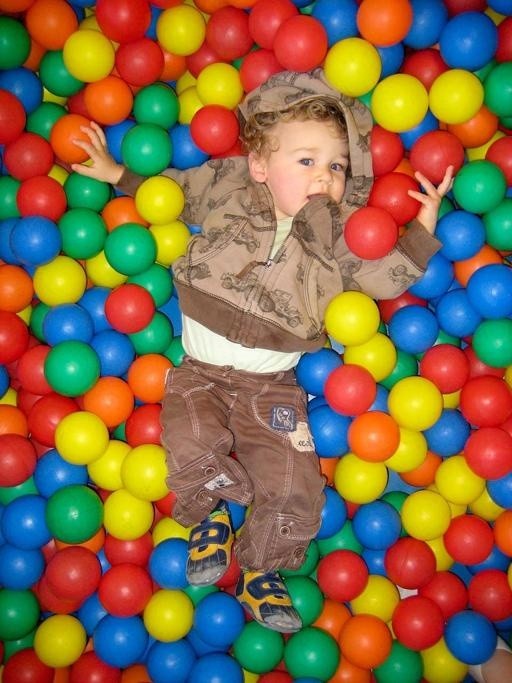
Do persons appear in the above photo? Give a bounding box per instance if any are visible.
[70,66,455,634]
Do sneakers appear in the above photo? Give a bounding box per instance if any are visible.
[186,510,235,586]
[235,571,302,633]
